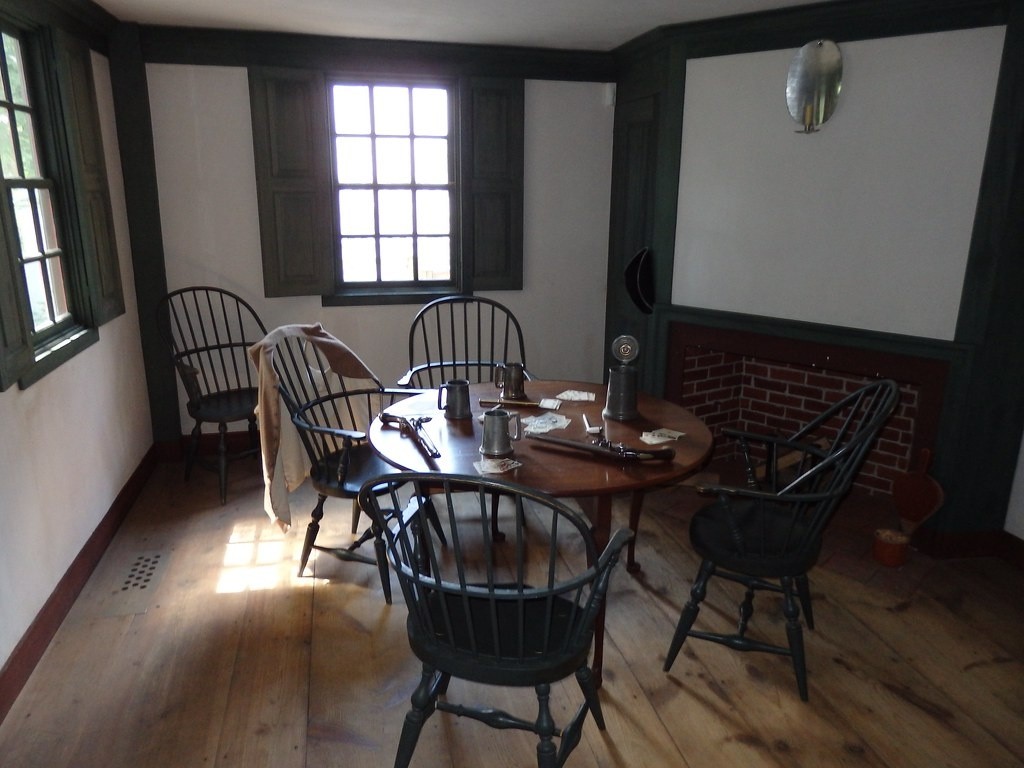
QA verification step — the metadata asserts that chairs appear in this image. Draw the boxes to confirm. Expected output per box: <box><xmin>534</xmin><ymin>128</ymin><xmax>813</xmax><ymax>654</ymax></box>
<box><xmin>155</xmin><ymin>287</ymin><xmax>268</xmax><ymax>505</ymax></box>
<box><xmin>397</xmin><ymin>296</ymin><xmax>542</xmax><ymax>527</ymax></box>
<box><xmin>661</xmin><ymin>380</ymin><xmax>902</xmax><ymax>703</ymax></box>
<box><xmin>356</xmin><ymin>473</ymin><xmax>636</xmax><ymax>768</ymax></box>
<box><xmin>248</xmin><ymin>322</ymin><xmax>448</xmax><ymax>606</ymax></box>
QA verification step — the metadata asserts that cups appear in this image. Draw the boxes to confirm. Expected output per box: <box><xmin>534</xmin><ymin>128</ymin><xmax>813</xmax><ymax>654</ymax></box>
<box><xmin>479</xmin><ymin>411</ymin><xmax>520</xmax><ymax>456</ymax></box>
<box><xmin>601</xmin><ymin>335</ymin><xmax>641</xmax><ymax>422</ymax></box>
<box><xmin>438</xmin><ymin>379</ymin><xmax>473</xmax><ymax>420</ymax></box>
<box><xmin>495</xmin><ymin>362</ymin><xmax>528</xmax><ymax>400</ymax></box>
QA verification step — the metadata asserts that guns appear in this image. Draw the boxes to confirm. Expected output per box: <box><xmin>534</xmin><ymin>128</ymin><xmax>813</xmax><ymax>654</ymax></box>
<box><xmin>526</xmin><ymin>431</ymin><xmax>676</xmax><ymax>465</ymax></box>
<box><xmin>377</xmin><ymin>412</ymin><xmax>440</xmax><ymax>473</ymax></box>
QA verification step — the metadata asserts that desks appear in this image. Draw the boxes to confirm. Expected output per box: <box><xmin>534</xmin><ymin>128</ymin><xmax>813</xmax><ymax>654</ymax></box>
<box><xmin>367</xmin><ymin>380</ymin><xmax>712</xmax><ymax>691</ymax></box>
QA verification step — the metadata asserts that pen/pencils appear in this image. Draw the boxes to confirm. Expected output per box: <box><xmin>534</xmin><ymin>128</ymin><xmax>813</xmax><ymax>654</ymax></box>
<box><xmin>478</xmin><ymin>399</ymin><xmax>539</xmax><ymax>408</ymax></box>
